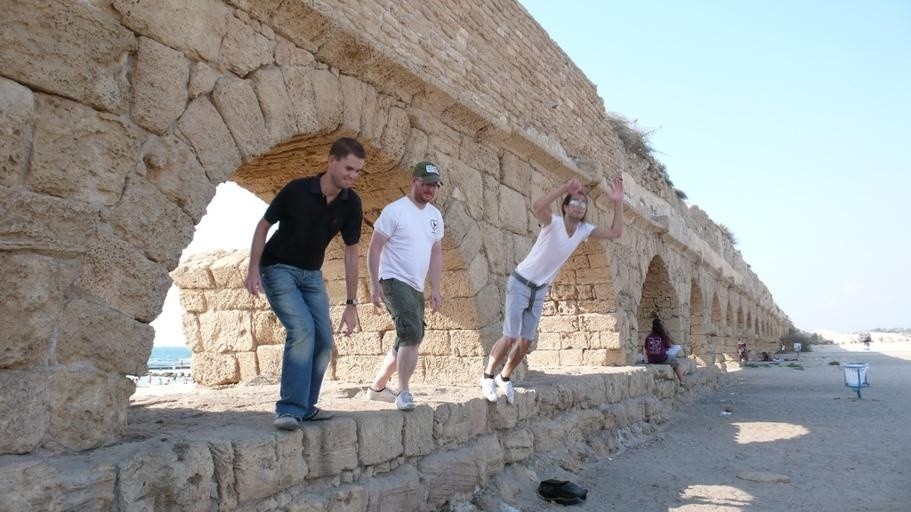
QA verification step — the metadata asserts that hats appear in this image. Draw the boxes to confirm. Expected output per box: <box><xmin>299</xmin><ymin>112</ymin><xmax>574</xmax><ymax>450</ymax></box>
<box><xmin>413</xmin><ymin>161</ymin><xmax>440</xmax><ymax>184</ymax></box>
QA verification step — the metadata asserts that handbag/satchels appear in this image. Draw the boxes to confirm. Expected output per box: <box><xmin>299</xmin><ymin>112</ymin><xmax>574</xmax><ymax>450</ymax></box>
<box><xmin>539</xmin><ymin>479</ymin><xmax>587</xmax><ymax>505</ymax></box>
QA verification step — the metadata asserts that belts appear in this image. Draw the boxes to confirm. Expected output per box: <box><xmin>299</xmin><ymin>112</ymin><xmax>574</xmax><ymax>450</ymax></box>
<box><xmin>512</xmin><ymin>270</ymin><xmax>547</xmax><ymax>311</ymax></box>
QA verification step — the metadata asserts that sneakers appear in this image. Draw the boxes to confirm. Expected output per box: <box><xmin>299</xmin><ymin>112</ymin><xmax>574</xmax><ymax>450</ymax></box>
<box><xmin>367</xmin><ymin>387</ymin><xmax>398</xmax><ymax>402</ymax></box>
<box><xmin>274</xmin><ymin>414</ymin><xmax>298</xmax><ymax>430</ymax></box>
<box><xmin>303</xmin><ymin>408</ymin><xmax>334</xmax><ymax>419</ymax></box>
<box><xmin>479</xmin><ymin>374</ymin><xmax>497</xmax><ymax>402</ymax></box>
<box><xmin>396</xmin><ymin>392</ymin><xmax>416</xmax><ymax>410</ymax></box>
<box><xmin>495</xmin><ymin>374</ymin><xmax>514</xmax><ymax>405</ymax></box>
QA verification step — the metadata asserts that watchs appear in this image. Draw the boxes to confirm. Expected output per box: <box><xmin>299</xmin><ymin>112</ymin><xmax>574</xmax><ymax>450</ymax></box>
<box><xmin>346</xmin><ymin>296</ymin><xmax>360</xmax><ymax>306</ymax></box>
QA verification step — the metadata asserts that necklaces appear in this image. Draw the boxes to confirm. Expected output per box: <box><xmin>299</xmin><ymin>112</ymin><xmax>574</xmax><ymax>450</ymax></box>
<box><xmin>565</xmin><ymin>216</ymin><xmax>579</xmax><ymax>237</ymax></box>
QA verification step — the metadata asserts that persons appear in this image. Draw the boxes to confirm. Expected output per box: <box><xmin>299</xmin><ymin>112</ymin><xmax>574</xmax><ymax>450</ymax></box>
<box><xmin>368</xmin><ymin>159</ymin><xmax>448</xmax><ymax>411</ymax></box>
<box><xmin>474</xmin><ymin>176</ymin><xmax>625</xmax><ymax>404</ymax></box>
<box><xmin>738</xmin><ymin>343</ymin><xmax>747</xmax><ymax>366</ymax></box>
<box><xmin>761</xmin><ymin>351</ymin><xmax>800</xmax><ymax>362</ymax></box>
<box><xmin>643</xmin><ymin>318</ymin><xmax>690</xmax><ymax>391</ymax></box>
<box><xmin>243</xmin><ymin>136</ymin><xmax>368</xmax><ymax>432</ymax></box>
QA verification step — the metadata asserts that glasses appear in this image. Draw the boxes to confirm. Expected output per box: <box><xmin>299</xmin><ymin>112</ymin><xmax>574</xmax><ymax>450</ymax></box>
<box><xmin>570</xmin><ymin>199</ymin><xmax>586</xmax><ymax>208</ymax></box>
